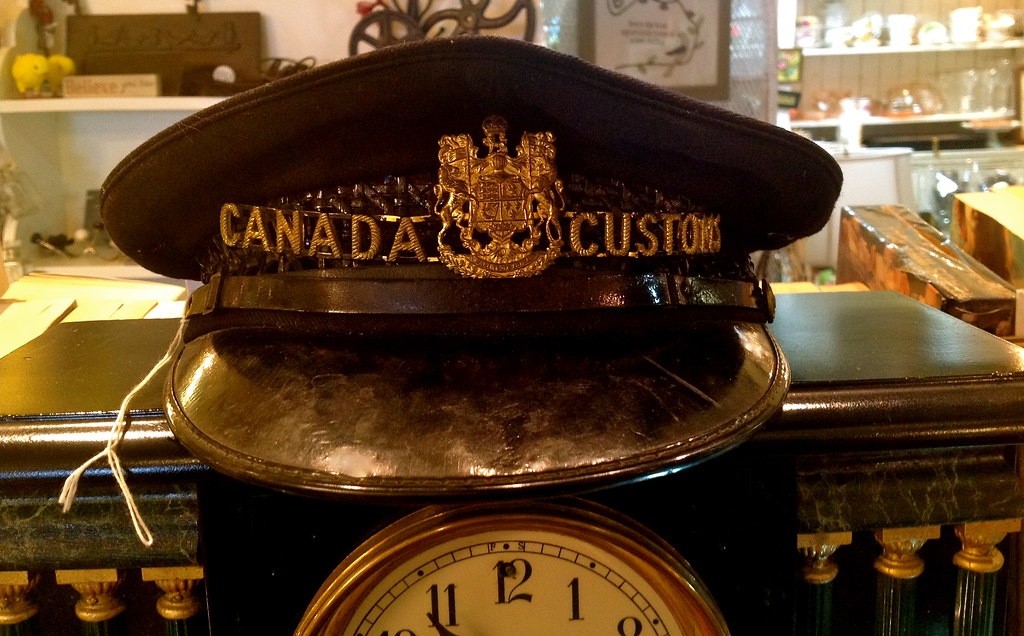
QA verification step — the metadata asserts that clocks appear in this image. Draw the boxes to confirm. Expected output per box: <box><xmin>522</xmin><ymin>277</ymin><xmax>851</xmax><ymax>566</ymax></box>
<box><xmin>294</xmin><ymin>494</ymin><xmax>732</xmax><ymax>636</ymax></box>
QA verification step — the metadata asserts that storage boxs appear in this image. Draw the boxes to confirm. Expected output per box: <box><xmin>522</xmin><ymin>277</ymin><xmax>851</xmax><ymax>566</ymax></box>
<box><xmin>837</xmin><ymin>204</ymin><xmax>1017</xmax><ymax>337</ymax></box>
<box><xmin>62</xmin><ymin>73</ymin><xmax>162</xmax><ymax>98</ymax></box>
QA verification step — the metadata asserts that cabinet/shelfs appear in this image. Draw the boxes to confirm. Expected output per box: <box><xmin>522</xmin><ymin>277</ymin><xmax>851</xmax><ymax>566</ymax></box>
<box><xmin>774</xmin><ymin>0</ymin><xmax>1024</xmax><ymax>128</ymax></box>
<box><xmin>0</xmin><ymin>6</ymin><xmax>233</xmax><ymax>280</ymax></box>
<box><xmin>869</xmin><ymin>134</ymin><xmax>1024</xmax><ymax>236</ymax></box>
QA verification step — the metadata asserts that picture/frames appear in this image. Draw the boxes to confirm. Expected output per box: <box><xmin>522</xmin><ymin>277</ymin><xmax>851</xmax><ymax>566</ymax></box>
<box><xmin>579</xmin><ymin>0</ymin><xmax>731</xmax><ymax>100</ymax></box>
